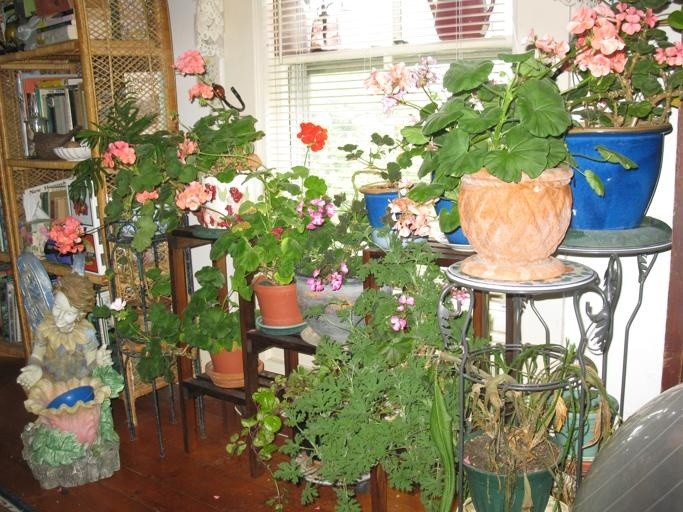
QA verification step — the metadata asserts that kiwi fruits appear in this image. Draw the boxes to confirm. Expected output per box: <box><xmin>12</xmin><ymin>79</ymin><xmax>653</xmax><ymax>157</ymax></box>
<box><xmin>63</xmin><ymin>142</ymin><xmax>80</xmax><ymax>148</ymax></box>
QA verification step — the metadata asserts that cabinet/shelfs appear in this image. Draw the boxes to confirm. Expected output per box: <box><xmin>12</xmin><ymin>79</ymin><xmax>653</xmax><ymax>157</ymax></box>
<box><xmin>0</xmin><ymin>0</ymin><xmax>191</xmax><ymax>428</ymax></box>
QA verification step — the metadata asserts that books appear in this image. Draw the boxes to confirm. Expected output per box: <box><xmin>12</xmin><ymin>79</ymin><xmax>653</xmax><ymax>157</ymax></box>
<box><xmin>22</xmin><ymin>177</ymin><xmax>73</xmax><ymax>231</ymax></box>
<box><xmin>6</xmin><ymin>283</ymin><xmax>22</xmax><ymax>344</ymax></box>
<box><xmin>95</xmin><ymin>288</ymin><xmax>113</xmax><ymax>348</ymax></box>
<box><xmin>24</xmin><ymin>9</ymin><xmax>111</xmax><ymax>49</ymax></box>
<box><xmin>16</xmin><ymin>71</ymin><xmax>166</xmax><ymax>158</ymax></box>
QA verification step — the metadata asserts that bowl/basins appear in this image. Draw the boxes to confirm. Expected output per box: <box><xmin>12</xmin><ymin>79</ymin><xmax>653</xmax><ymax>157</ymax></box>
<box><xmin>49</xmin><ymin>385</ymin><xmax>94</xmax><ymax>409</ymax></box>
<box><xmin>52</xmin><ymin>148</ymin><xmax>91</xmax><ymax>162</ymax></box>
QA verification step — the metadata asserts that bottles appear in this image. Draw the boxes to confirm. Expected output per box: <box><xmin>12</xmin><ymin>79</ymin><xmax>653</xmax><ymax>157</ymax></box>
<box><xmin>25</xmin><ymin>112</ymin><xmax>48</xmax><ymax>159</ymax></box>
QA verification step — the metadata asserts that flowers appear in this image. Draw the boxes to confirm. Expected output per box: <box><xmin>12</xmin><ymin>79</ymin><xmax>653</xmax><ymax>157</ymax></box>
<box><xmin>366</xmin><ymin>270</ymin><xmax>481</xmax><ymax>347</ymax></box>
<box><xmin>361</xmin><ymin>51</ymin><xmax>456</xmax><ymax>130</ymax></box>
<box><xmin>49</xmin><ymin>48</ymin><xmax>258</xmax><ymax>266</ymax></box>
<box><xmin>529</xmin><ymin>0</ymin><xmax>683</xmax><ymax>127</ymax></box>
<box><xmin>222</xmin><ymin>118</ymin><xmax>353</xmax><ymax>294</ymax></box>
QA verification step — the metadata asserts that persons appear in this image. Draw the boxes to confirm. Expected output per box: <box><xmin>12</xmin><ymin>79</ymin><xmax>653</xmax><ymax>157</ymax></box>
<box><xmin>17</xmin><ymin>274</ymin><xmax>115</xmax><ymax>391</ymax></box>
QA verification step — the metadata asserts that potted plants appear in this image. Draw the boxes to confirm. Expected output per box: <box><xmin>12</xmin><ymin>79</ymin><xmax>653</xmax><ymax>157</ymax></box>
<box><xmin>454</xmin><ymin>355</ymin><xmax>568</xmax><ymax>511</ymax></box>
<box><xmin>421</xmin><ymin>48</ymin><xmax>577</xmax><ymax>282</ymax></box>
<box><xmin>150</xmin><ymin>267</ymin><xmax>260</xmax><ymax>388</ymax></box>
<box><xmin>345</xmin><ymin>131</ymin><xmax>418</xmax><ymax>229</ymax></box>
